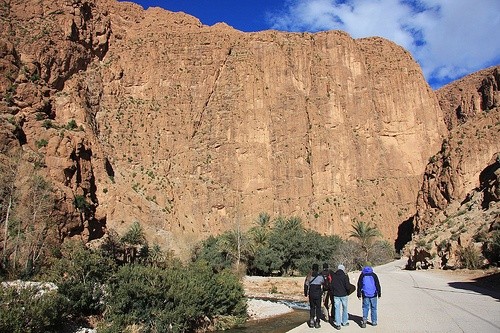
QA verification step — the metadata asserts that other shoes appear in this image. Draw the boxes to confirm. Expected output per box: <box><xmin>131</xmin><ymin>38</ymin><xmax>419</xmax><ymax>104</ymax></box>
<box><xmin>372</xmin><ymin>323</ymin><xmax>377</xmax><ymax>326</ymax></box>
<box><xmin>342</xmin><ymin>322</ymin><xmax>349</xmax><ymax>326</ymax></box>
<box><xmin>336</xmin><ymin>325</ymin><xmax>341</xmax><ymax>330</ymax></box>
<box><xmin>307</xmin><ymin>320</ymin><xmax>321</xmax><ymax>328</ymax></box>
<box><xmin>361</xmin><ymin>319</ymin><xmax>368</xmax><ymax>325</ymax></box>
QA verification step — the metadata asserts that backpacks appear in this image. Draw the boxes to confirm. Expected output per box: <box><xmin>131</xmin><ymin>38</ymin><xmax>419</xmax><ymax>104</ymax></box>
<box><xmin>322</xmin><ymin>274</ymin><xmax>330</xmax><ymax>291</ymax></box>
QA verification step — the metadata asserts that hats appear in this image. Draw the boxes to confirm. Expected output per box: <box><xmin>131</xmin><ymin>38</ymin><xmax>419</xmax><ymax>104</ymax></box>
<box><xmin>363</xmin><ymin>261</ymin><xmax>373</xmax><ymax>267</ymax></box>
<box><xmin>338</xmin><ymin>264</ymin><xmax>346</xmax><ymax>272</ymax></box>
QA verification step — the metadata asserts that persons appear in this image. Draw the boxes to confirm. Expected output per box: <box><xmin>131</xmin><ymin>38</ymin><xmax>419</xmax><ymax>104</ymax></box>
<box><xmin>305</xmin><ymin>263</ymin><xmax>323</xmax><ymax>327</ymax></box>
<box><xmin>330</xmin><ymin>264</ymin><xmax>356</xmax><ymax>330</ymax></box>
<box><xmin>357</xmin><ymin>261</ymin><xmax>382</xmax><ymax>328</ymax></box>
<box><xmin>320</xmin><ymin>263</ymin><xmax>335</xmax><ymax>323</ymax></box>
